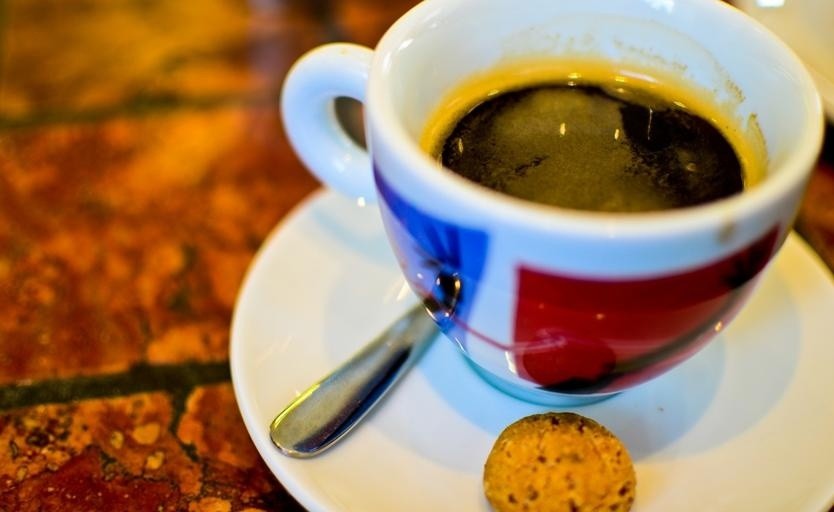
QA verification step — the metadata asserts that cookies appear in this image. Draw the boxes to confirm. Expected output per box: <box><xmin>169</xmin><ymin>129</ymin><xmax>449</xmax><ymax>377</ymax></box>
<box><xmin>483</xmin><ymin>412</ymin><xmax>636</xmax><ymax>512</ymax></box>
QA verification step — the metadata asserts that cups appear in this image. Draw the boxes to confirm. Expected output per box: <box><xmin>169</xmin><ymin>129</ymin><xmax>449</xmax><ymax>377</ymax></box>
<box><xmin>276</xmin><ymin>0</ymin><xmax>828</xmax><ymax>411</ymax></box>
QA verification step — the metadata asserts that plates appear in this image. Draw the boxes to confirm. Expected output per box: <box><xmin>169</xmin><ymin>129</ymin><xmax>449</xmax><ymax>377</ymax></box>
<box><xmin>225</xmin><ymin>182</ymin><xmax>834</xmax><ymax>512</ymax></box>
